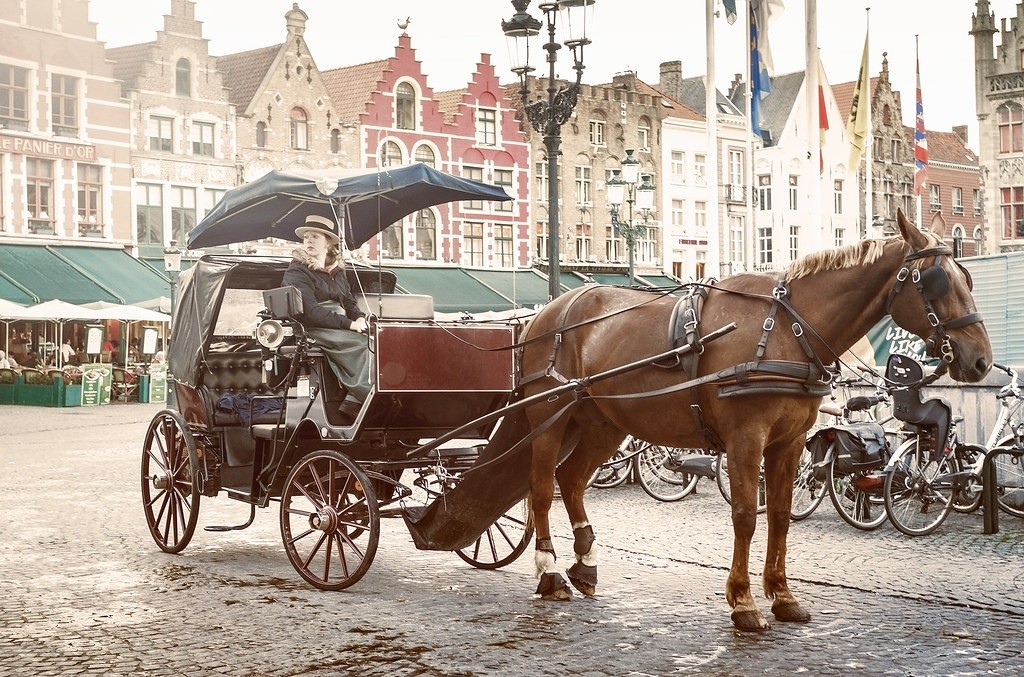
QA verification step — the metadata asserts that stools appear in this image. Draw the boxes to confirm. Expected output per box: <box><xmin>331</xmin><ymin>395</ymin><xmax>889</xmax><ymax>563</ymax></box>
<box><xmin>252</xmin><ymin>424</ymin><xmax>284</xmax><ymax>440</ymax></box>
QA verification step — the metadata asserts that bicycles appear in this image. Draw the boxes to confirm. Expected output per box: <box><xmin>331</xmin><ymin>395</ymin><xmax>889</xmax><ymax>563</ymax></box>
<box><xmin>548</xmin><ymin>361</ymin><xmax>985</xmax><ymax>537</ymax></box>
<box><xmin>881</xmin><ymin>363</ymin><xmax>1024</xmax><ymax>536</ymax></box>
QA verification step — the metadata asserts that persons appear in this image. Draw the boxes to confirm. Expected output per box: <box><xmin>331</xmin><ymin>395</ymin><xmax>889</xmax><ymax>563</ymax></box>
<box><xmin>48</xmin><ymin>337</ymin><xmax>89</xmax><ymax>367</ymax></box>
<box><xmin>102</xmin><ymin>337</ymin><xmax>139</xmax><ymax>352</ymax></box>
<box><xmin>281</xmin><ymin>215</ymin><xmax>375</xmax><ymax>428</ymax></box>
<box><xmin>0</xmin><ymin>349</ymin><xmax>19</xmax><ymax>368</ymax></box>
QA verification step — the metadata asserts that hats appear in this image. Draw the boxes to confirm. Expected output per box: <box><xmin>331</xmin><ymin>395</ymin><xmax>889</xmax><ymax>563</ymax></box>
<box><xmin>294</xmin><ymin>215</ymin><xmax>339</xmax><ymax>245</ymax></box>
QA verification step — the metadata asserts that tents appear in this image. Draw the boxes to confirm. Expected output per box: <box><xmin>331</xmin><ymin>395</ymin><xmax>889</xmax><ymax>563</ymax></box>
<box><xmin>0</xmin><ymin>295</ymin><xmax>173</xmax><ymax>403</ymax></box>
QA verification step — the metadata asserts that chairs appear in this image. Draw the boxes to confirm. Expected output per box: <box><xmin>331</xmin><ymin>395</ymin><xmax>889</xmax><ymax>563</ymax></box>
<box><xmin>0</xmin><ymin>351</ymin><xmax>138</xmax><ymax>404</ymax></box>
<box><xmin>884</xmin><ymin>353</ymin><xmax>952</xmax><ymax>462</ymax></box>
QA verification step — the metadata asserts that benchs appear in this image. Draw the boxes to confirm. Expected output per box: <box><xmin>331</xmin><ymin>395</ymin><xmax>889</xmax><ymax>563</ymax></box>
<box><xmin>200</xmin><ymin>353</ymin><xmax>316</xmax><ymax>425</ymax></box>
<box><xmin>261</xmin><ymin>287</ymin><xmax>434</xmax><ymax>347</ymax></box>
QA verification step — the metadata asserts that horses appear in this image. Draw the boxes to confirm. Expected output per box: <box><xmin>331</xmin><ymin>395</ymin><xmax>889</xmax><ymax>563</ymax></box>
<box><xmin>516</xmin><ymin>207</ymin><xmax>993</xmax><ymax>633</ymax></box>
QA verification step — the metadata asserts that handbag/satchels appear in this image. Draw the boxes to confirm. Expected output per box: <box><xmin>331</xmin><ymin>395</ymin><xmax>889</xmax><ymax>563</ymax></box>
<box><xmin>806</xmin><ymin>421</ymin><xmax>889</xmax><ymax>480</ymax></box>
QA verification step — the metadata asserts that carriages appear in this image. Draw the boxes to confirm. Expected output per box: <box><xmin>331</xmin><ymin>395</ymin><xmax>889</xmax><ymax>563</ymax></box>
<box><xmin>139</xmin><ymin>162</ymin><xmax>994</xmax><ymax>634</ymax></box>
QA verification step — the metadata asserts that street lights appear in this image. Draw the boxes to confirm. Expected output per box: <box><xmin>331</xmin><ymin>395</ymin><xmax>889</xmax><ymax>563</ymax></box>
<box><xmin>604</xmin><ymin>148</ymin><xmax>657</xmax><ymax>287</ymax></box>
<box><xmin>501</xmin><ymin>0</ymin><xmax>595</xmax><ymax>302</ymax></box>
<box><xmin>163</xmin><ymin>238</ymin><xmax>182</xmax><ymax>333</ymax></box>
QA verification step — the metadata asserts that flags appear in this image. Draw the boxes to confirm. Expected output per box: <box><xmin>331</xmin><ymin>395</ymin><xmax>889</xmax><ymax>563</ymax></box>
<box><xmin>723</xmin><ymin>0</ymin><xmax>738</xmax><ymax>25</ymax></box>
<box><xmin>914</xmin><ymin>54</ymin><xmax>928</xmax><ymax>197</ymax></box>
<box><xmin>846</xmin><ymin>30</ymin><xmax>868</xmax><ymax>174</ymax></box>
<box><xmin>817</xmin><ymin>59</ymin><xmax>829</xmax><ymax>176</ymax></box>
<box><xmin>750</xmin><ymin>0</ymin><xmax>785</xmax><ymax>141</ymax></box>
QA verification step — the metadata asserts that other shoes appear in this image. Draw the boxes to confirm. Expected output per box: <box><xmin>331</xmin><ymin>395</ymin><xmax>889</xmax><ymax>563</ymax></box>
<box><xmin>338</xmin><ymin>401</ymin><xmax>362</xmax><ymax>419</ymax></box>
